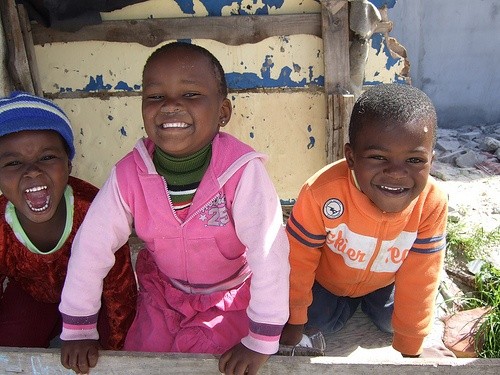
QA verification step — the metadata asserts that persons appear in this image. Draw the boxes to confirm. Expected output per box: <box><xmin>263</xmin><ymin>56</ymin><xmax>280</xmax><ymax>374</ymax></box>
<box><xmin>278</xmin><ymin>83</ymin><xmax>448</xmax><ymax>358</ymax></box>
<box><xmin>0</xmin><ymin>90</ymin><xmax>138</xmax><ymax>350</ymax></box>
<box><xmin>59</xmin><ymin>42</ymin><xmax>291</xmax><ymax>375</ymax></box>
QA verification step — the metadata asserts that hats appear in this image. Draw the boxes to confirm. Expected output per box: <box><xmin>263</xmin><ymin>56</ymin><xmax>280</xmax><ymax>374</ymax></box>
<box><xmin>0</xmin><ymin>90</ymin><xmax>76</xmax><ymax>161</ymax></box>
